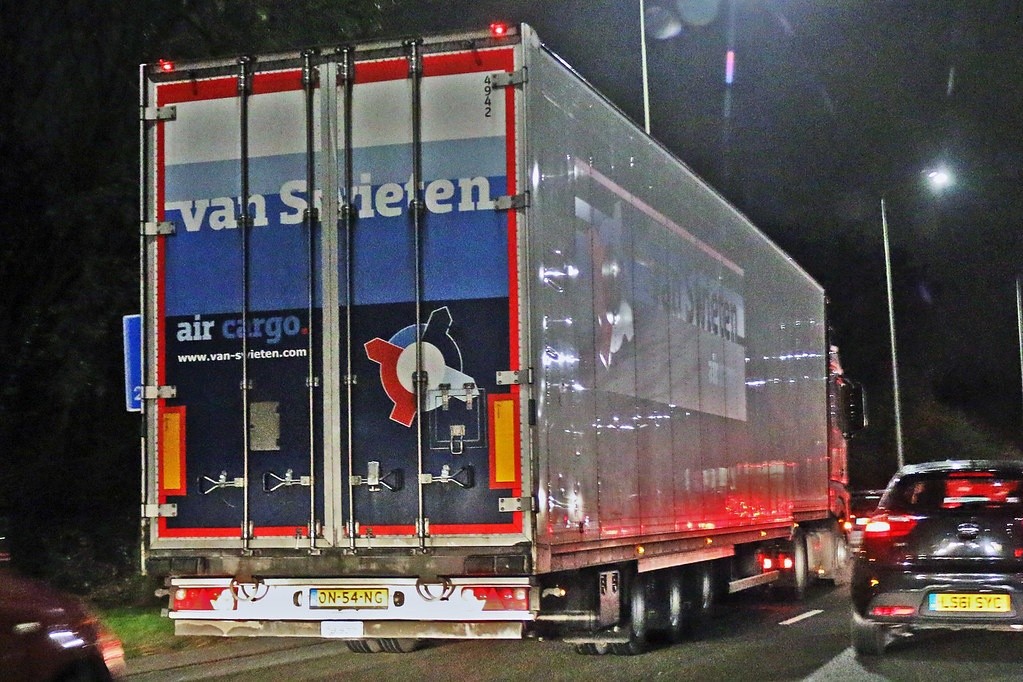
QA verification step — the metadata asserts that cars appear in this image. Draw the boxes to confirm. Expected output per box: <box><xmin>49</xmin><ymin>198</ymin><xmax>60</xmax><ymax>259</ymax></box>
<box><xmin>839</xmin><ymin>488</ymin><xmax>889</xmax><ymax>547</ymax></box>
<box><xmin>0</xmin><ymin>550</ymin><xmax>127</xmax><ymax>682</ymax></box>
<box><xmin>849</xmin><ymin>458</ymin><xmax>1023</xmax><ymax>660</ymax></box>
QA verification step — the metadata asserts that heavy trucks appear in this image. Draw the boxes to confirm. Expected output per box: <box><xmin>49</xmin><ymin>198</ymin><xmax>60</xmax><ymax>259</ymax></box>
<box><xmin>137</xmin><ymin>22</ymin><xmax>870</xmax><ymax>658</ymax></box>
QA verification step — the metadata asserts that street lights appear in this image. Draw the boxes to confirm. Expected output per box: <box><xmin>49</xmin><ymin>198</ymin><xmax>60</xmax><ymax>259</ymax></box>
<box><xmin>879</xmin><ymin>160</ymin><xmax>957</xmax><ymax>471</ymax></box>
